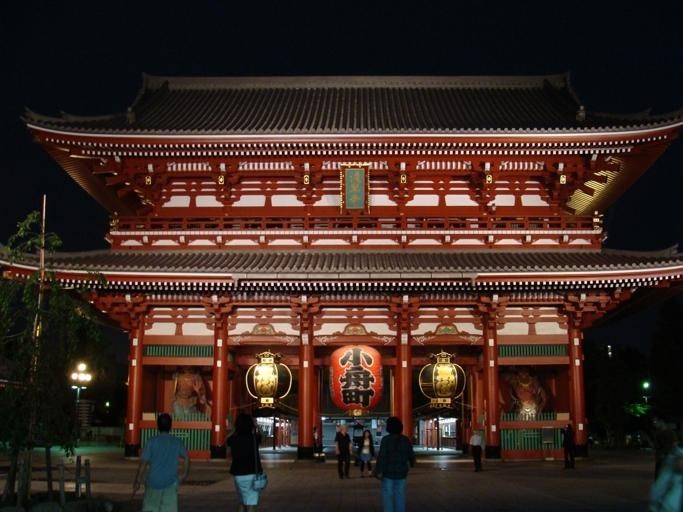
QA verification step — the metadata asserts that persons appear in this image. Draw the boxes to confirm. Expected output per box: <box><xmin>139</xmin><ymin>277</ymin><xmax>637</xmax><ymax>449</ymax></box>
<box><xmin>509</xmin><ymin>365</ymin><xmax>548</xmax><ymax>419</ymax></box>
<box><xmin>648</xmin><ymin>421</ymin><xmax>683</xmax><ymax>512</ymax></box>
<box><xmin>334</xmin><ymin>425</ymin><xmax>353</xmax><ymax>480</ymax></box>
<box><xmin>563</xmin><ymin>424</ymin><xmax>575</xmax><ymax>470</ymax></box>
<box><xmin>651</xmin><ymin>414</ymin><xmax>675</xmax><ymax>481</ymax></box>
<box><xmin>370</xmin><ymin>417</ymin><xmax>417</xmax><ymax>512</ymax></box>
<box><xmin>470</xmin><ymin>430</ymin><xmax>482</xmax><ymax>472</ymax></box>
<box><xmin>357</xmin><ymin>430</ymin><xmax>375</xmax><ymax>477</ymax></box>
<box><xmin>134</xmin><ymin>413</ymin><xmax>190</xmax><ymax>512</ymax></box>
<box><xmin>171</xmin><ymin>366</ymin><xmax>206</xmax><ymax>416</ymax></box>
<box><xmin>227</xmin><ymin>413</ymin><xmax>265</xmax><ymax>512</ymax></box>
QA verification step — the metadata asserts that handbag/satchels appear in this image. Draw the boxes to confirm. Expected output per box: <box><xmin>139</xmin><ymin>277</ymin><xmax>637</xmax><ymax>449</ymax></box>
<box><xmin>252</xmin><ymin>474</ymin><xmax>267</xmax><ymax>490</ymax></box>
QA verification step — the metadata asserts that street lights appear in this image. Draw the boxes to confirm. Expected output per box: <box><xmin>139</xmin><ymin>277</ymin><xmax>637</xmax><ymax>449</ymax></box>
<box><xmin>71</xmin><ymin>363</ymin><xmax>92</xmax><ymax>448</ymax></box>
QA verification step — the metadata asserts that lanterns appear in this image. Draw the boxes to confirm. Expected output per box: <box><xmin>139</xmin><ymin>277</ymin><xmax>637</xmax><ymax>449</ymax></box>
<box><xmin>418</xmin><ymin>352</ymin><xmax>466</xmax><ymax>410</ymax></box>
<box><xmin>329</xmin><ymin>344</ymin><xmax>384</xmax><ymax>417</ymax></box>
<box><xmin>245</xmin><ymin>352</ymin><xmax>292</xmax><ymax>409</ymax></box>
